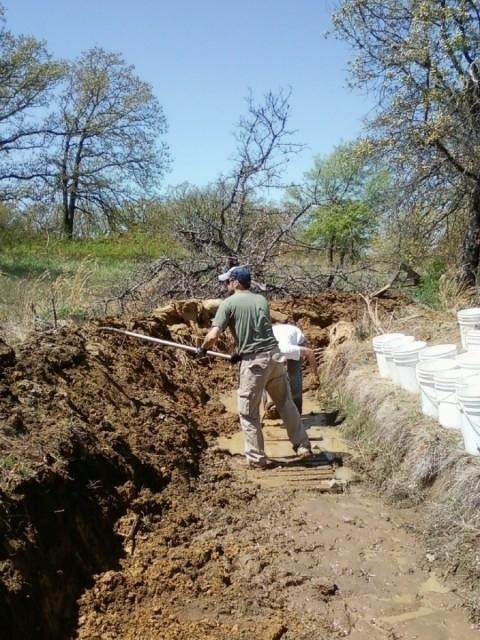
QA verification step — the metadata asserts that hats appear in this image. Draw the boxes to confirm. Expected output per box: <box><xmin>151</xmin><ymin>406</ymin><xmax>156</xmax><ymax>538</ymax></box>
<box><xmin>218</xmin><ymin>267</ymin><xmax>250</xmax><ymax>284</ymax></box>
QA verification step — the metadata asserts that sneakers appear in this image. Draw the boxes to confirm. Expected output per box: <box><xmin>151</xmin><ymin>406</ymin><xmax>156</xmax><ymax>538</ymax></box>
<box><xmin>238</xmin><ymin>460</ymin><xmax>265</xmax><ymax>470</ymax></box>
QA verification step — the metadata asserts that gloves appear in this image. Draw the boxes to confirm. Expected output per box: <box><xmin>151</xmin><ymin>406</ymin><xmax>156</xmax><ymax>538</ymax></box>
<box><xmin>230</xmin><ymin>354</ymin><xmax>241</xmax><ymax>364</ymax></box>
<box><xmin>197</xmin><ymin>346</ymin><xmax>207</xmax><ymax>358</ymax></box>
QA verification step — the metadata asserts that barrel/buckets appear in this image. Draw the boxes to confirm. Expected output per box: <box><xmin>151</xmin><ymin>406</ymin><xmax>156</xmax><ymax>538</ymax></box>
<box><xmin>369</xmin><ymin>308</ymin><xmax>480</xmax><ymax>456</ymax></box>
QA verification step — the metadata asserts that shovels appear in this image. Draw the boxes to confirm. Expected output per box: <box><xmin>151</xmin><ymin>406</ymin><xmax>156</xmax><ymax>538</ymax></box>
<box><xmin>69</xmin><ymin>314</ymin><xmax>232</xmax><ymax>361</ymax></box>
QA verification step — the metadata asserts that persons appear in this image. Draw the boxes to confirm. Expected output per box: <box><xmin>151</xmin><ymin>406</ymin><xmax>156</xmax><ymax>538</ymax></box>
<box><xmin>195</xmin><ymin>266</ymin><xmax>313</xmax><ymax>470</ymax></box>
<box><xmin>271</xmin><ymin>323</ymin><xmax>320</xmax><ymax>415</ymax></box>
